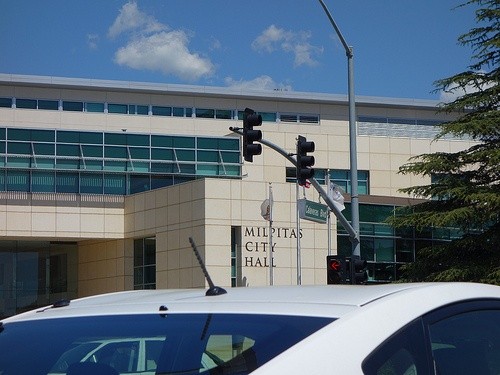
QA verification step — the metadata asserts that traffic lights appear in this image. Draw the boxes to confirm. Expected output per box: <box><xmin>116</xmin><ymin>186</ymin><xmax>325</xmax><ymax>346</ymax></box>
<box><xmin>349</xmin><ymin>255</ymin><xmax>368</xmax><ymax>285</ymax></box>
<box><xmin>327</xmin><ymin>254</ymin><xmax>347</xmax><ymax>284</ymax></box>
<box><xmin>241</xmin><ymin>107</ymin><xmax>263</xmax><ymax>162</ymax></box>
<box><xmin>295</xmin><ymin>135</ymin><xmax>315</xmax><ymax>186</ymax></box>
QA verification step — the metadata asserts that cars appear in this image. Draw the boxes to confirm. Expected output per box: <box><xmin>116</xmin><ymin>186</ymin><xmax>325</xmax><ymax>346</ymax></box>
<box><xmin>0</xmin><ymin>285</ymin><xmax>500</xmax><ymax>375</ymax></box>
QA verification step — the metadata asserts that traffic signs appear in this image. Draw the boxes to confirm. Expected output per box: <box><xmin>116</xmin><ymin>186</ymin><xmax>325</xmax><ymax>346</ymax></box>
<box><xmin>298</xmin><ymin>198</ymin><xmax>328</xmax><ymax>224</ymax></box>
